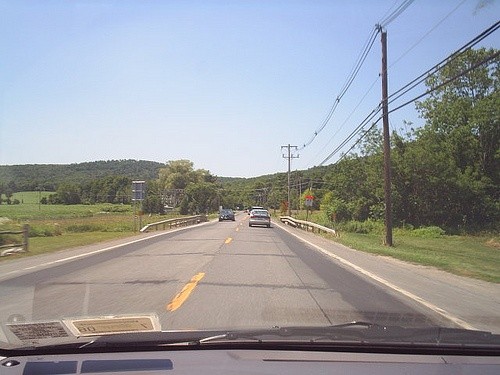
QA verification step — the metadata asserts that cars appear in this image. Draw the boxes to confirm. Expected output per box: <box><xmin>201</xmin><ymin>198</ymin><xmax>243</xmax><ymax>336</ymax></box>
<box><xmin>248</xmin><ymin>208</ymin><xmax>271</xmax><ymax>227</ymax></box>
<box><xmin>218</xmin><ymin>208</ymin><xmax>234</xmax><ymax>221</ymax></box>
